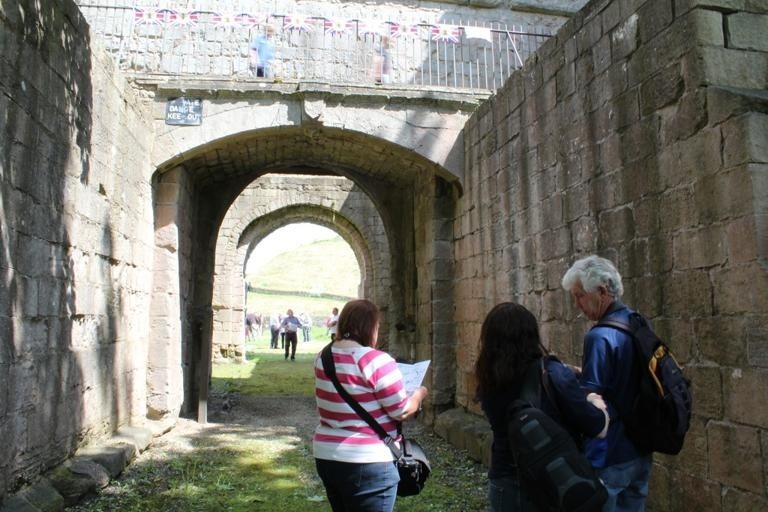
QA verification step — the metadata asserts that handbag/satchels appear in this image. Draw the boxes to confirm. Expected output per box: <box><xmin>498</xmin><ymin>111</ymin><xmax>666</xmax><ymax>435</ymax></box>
<box><xmin>389</xmin><ymin>434</ymin><xmax>432</xmax><ymax>498</ymax></box>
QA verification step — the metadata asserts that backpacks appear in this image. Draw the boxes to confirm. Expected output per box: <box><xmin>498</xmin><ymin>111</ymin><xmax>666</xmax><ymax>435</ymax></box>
<box><xmin>589</xmin><ymin>311</ymin><xmax>695</xmax><ymax>458</ymax></box>
<box><xmin>495</xmin><ymin>354</ymin><xmax>611</xmax><ymax>511</ymax></box>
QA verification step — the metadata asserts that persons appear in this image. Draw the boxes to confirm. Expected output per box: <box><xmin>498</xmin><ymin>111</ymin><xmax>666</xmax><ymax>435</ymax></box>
<box><xmin>472</xmin><ymin>302</ymin><xmax>609</xmax><ymax>510</ymax></box>
<box><xmin>559</xmin><ymin>253</ymin><xmax>692</xmax><ymax>512</ymax></box>
<box><xmin>244</xmin><ymin>312</ymin><xmax>312</xmax><ymax>350</ymax></box>
<box><xmin>275</xmin><ymin>309</ymin><xmax>302</xmax><ymax>361</ymax></box>
<box><xmin>312</xmin><ymin>297</ymin><xmax>430</xmax><ymax>512</ymax></box>
<box><xmin>327</xmin><ymin>307</ymin><xmax>339</xmax><ymax>341</ymax></box>
